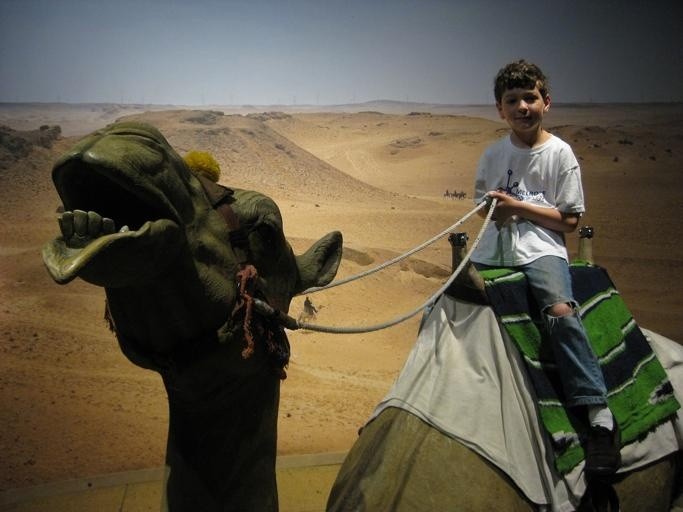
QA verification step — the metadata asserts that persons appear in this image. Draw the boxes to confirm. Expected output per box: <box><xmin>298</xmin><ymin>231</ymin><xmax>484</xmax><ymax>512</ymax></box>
<box><xmin>467</xmin><ymin>59</ymin><xmax>625</xmax><ymax>479</ymax></box>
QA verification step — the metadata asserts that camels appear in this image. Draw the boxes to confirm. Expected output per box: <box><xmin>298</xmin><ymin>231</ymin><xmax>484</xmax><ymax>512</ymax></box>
<box><xmin>40</xmin><ymin>119</ymin><xmax>682</xmax><ymax>511</ymax></box>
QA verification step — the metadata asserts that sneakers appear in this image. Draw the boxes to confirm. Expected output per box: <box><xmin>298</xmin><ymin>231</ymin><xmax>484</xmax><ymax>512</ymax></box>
<box><xmin>585</xmin><ymin>413</ymin><xmax>622</xmax><ymax>476</ymax></box>
